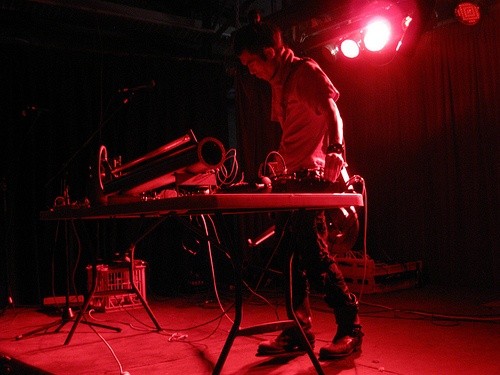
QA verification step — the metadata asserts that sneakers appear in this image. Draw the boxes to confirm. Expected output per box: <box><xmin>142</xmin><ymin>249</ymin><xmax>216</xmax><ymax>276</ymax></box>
<box><xmin>319</xmin><ymin>334</ymin><xmax>363</xmax><ymax>357</ymax></box>
<box><xmin>256</xmin><ymin>333</ymin><xmax>315</xmax><ymax>356</ymax></box>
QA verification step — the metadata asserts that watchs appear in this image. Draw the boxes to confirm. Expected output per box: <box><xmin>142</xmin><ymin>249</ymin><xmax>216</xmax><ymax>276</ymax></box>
<box><xmin>327</xmin><ymin>143</ymin><xmax>345</xmax><ymax>155</ymax></box>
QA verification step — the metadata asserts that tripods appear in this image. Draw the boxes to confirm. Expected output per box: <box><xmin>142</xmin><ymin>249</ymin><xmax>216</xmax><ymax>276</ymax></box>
<box><xmin>15</xmin><ymin>220</ymin><xmax>121</xmax><ymax>340</ymax></box>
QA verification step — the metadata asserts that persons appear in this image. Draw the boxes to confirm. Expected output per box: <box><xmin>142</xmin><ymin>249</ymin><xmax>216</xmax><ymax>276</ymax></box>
<box><xmin>230</xmin><ymin>21</ymin><xmax>365</xmax><ymax>359</ymax></box>
